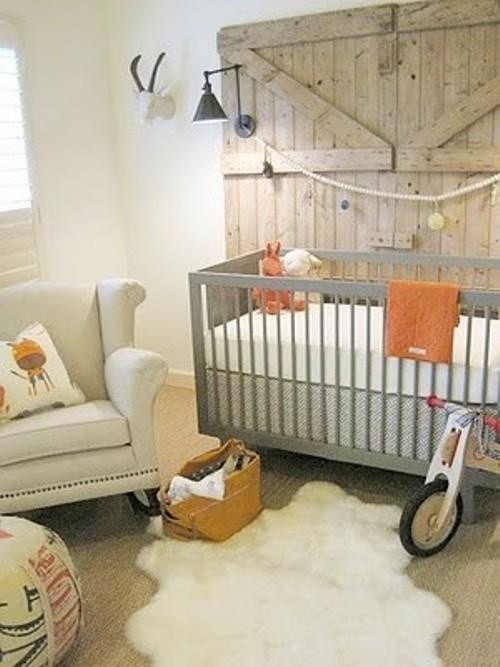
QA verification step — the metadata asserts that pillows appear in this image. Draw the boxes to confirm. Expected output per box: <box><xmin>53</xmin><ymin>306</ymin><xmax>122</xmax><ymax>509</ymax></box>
<box><xmin>1</xmin><ymin>321</ymin><xmax>85</xmax><ymax>424</ymax></box>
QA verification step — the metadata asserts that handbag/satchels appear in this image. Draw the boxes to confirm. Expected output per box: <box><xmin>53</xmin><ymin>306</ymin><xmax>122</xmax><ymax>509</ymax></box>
<box><xmin>157</xmin><ymin>438</ymin><xmax>264</xmax><ymax>543</ymax></box>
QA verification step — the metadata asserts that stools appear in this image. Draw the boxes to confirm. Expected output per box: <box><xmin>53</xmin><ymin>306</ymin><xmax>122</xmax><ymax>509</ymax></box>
<box><xmin>0</xmin><ymin>515</ymin><xmax>83</xmax><ymax>665</ymax></box>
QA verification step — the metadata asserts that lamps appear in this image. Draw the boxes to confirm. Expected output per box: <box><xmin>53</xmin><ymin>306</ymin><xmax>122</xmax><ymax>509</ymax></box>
<box><xmin>192</xmin><ymin>64</ymin><xmax>256</xmax><ymax>137</ymax></box>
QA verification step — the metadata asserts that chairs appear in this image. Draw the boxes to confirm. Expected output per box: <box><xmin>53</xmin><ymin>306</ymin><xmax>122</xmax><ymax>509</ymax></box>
<box><xmin>1</xmin><ymin>273</ymin><xmax>167</xmax><ymax>516</ymax></box>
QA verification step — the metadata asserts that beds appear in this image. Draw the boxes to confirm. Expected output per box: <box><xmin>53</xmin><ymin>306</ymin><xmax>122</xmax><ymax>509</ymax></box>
<box><xmin>188</xmin><ymin>243</ymin><xmax>500</xmax><ymax>524</ymax></box>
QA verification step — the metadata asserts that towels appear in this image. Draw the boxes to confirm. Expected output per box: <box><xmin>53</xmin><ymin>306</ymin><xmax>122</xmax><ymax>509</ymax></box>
<box><xmin>382</xmin><ymin>279</ymin><xmax>460</xmax><ymax>364</ymax></box>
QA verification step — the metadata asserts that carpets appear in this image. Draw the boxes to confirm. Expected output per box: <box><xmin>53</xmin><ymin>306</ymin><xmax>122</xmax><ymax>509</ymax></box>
<box><xmin>125</xmin><ymin>481</ymin><xmax>453</xmax><ymax>664</ymax></box>
